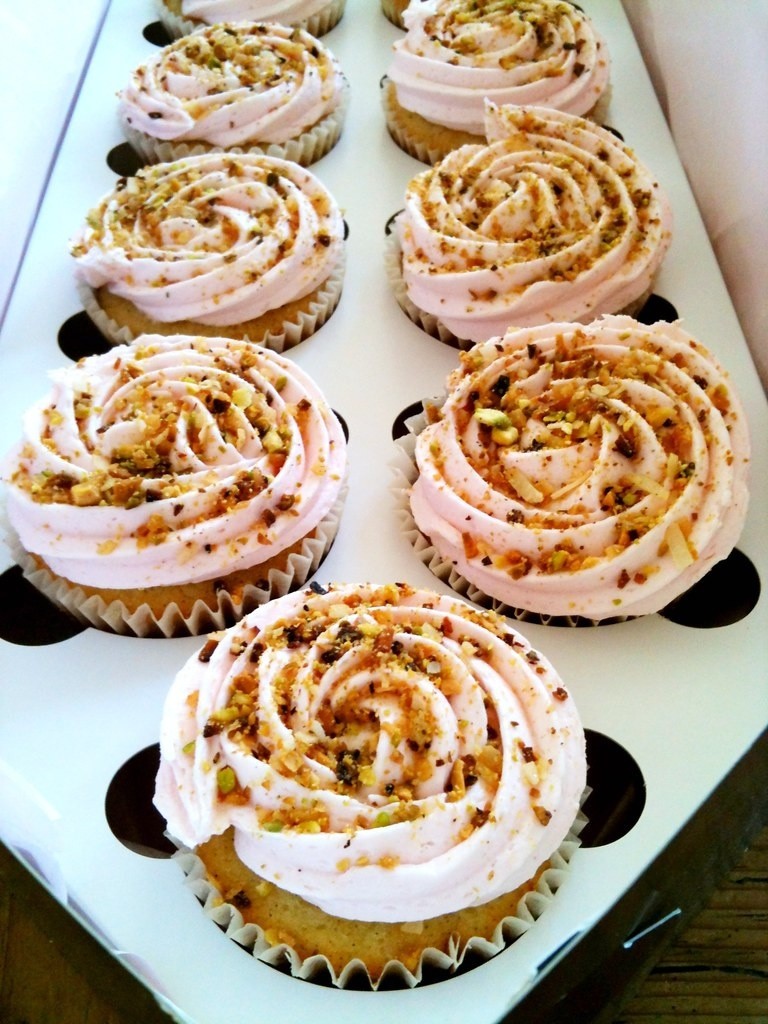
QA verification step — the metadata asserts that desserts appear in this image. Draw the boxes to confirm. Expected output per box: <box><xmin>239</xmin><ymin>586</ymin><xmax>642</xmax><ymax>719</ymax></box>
<box><xmin>0</xmin><ymin>0</ymin><xmax>752</xmax><ymax>988</ymax></box>
